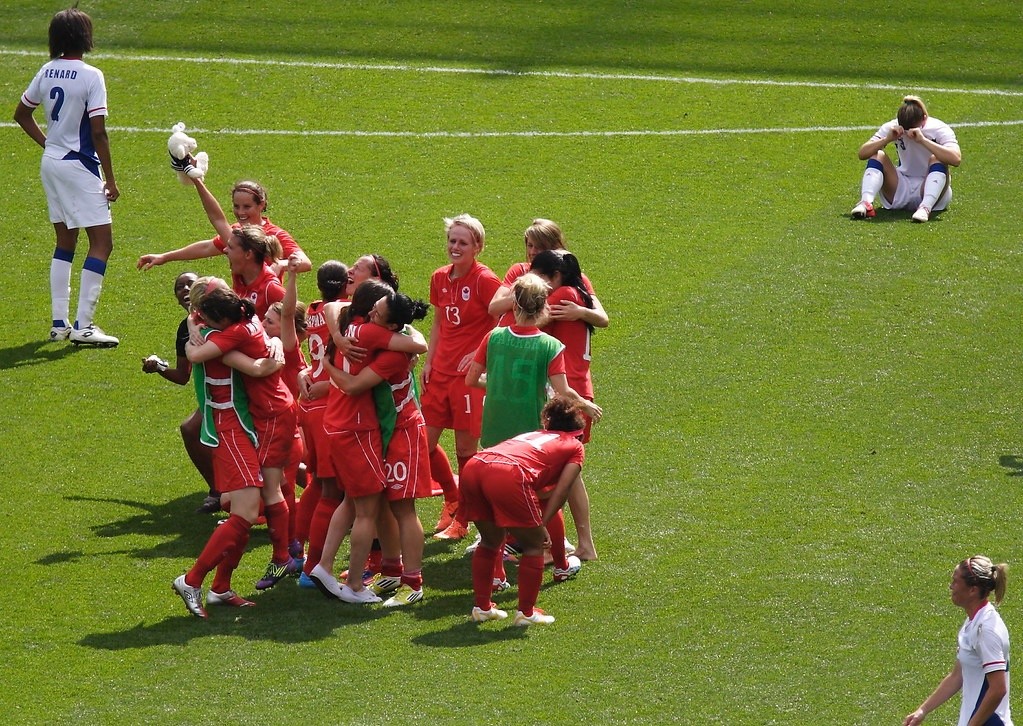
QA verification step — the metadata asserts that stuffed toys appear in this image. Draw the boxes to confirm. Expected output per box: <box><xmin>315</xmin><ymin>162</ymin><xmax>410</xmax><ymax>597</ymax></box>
<box><xmin>168</xmin><ymin>122</ymin><xmax>209</xmax><ymax>184</ymax></box>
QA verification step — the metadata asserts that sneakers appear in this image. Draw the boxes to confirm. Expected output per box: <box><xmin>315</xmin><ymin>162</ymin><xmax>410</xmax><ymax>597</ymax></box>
<box><xmin>912</xmin><ymin>208</ymin><xmax>929</xmax><ymax>222</ymax></box>
<box><xmin>67</xmin><ymin>327</ymin><xmax>120</xmax><ymax>349</ymax></box>
<box><xmin>513</xmin><ymin>609</ymin><xmax>555</xmax><ymax>627</ymax></box>
<box><xmin>171</xmin><ymin>573</ymin><xmax>208</xmax><ymax>619</ymax></box>
<box><xmin>367</xmin><ymin>573</ymin><xmax>403</xmax><ymax>595</ymax></box>
<box><xmin>466</xmin><ymin>534</ymin><xmax>483</xmax><ymax>553</ymax></box>
<box><xmin>553</xmin><ymin>555</ymin><xmax>581</xmax><ymax>581</ymax></box>
<box><xmin>472</xmin><ymin>607</ymin><xmax>508</xmax><ymax>622</ymax></box>
<box><xmin>435</xmin><ymin>501</ymin><xmax>459</xmax><ymax>531</ymax></box>
<box><xmin>851</xmin><ymin>201</ymin><xmax>874</xmax><ymax>219</ymax></box>
<box><xmin>255</xmin><ymin>555</ymin><xmax>297</xmax><ymax>589</ymax></box>
<box><xmin>206</xmin><ymin>589</ymin><xmax>256</xmax><ymax>607</ymax></box>
<box><xmin>382</xmin><ymin>583</ymin><xmax>423</xmax><ymax>609</ymax></box>
<box><xmin>195</xmin><ymin>495</ymin><xmax>223</xmax><ymax>512</ymax></box>
<box><xmin>337</xmin><ymin>583</ymin><xmax>384</xmax><ymax>604</ymax></box>
<box><xmin>310</xmin><ymin>564</ymin><xmax>340</xmax><ymax>597</ymax></box>
<box><xmin>491</xmin><ymin>578</ymin><xmax>510</xmax><ymax>591</ymax></box>
<box><xmin>52</xmin><ymin>325</ymin><xmax>71</xmax><ymax>339</ymax></box>
<box><xmin>433</xmin><ymin>519</ymin><xmax>471</xmax><ymax>540</ymax></box>
<box><xmin>288</xmin><ymin>540</ymin><xmax>303</xmax><ymax>558</ymax></box>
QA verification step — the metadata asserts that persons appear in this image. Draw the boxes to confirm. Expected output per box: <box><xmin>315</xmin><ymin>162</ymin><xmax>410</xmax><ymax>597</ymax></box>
<box><xmin>851</xmin><ymin>95</ymin><xmax>961</xmax><ymax>222</ymax></box>
<box><xmin>904</xmin><ymin>555</ymin><xmax>1014</xmax><ymax>726</ymax></box>
<box><xmin>13</xmin><ymin>8</ymin><xmax>120</xmax><ymax>347</ymax></box>
<box><xmin>137</xmin><ymin>153</ymin><xmax>609</xmax><ymax>626</ymax></box>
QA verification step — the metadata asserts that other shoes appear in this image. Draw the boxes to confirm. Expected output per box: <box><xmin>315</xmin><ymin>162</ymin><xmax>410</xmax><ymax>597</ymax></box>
<box><xmin>339</xmin><ymin>564</ymin><xmax>380</xmax><ymax>584</ymax></box>
<box><xmin>291</xmin><ymin>557</ymin><xmax>307</xmax><ymax>575</ymax></box>
<box><xmin>299</xmin><ymin>571</ymin><xmax>315</xmax><ymax>588</ymax></box>
